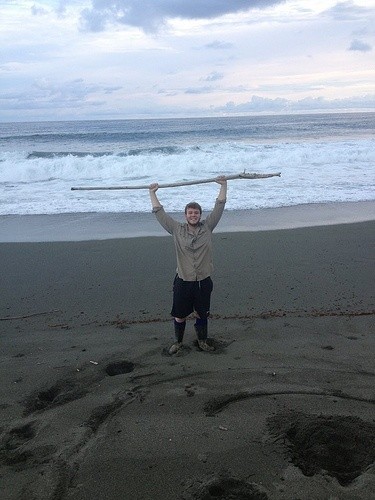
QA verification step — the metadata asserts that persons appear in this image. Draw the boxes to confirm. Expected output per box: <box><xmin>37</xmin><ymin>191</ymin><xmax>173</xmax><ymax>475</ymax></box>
<box><xmin>148</xmin><ymin>173</ymin><xmax>227</xmax><ymax>355</ymax></box>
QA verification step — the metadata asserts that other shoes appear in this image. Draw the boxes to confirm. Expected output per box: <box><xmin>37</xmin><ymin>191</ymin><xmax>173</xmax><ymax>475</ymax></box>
<box><xmin>169</xmin><ymin>343</ymin><xmax>184</xmax><ymax>355</ymax></box>
<box><xmin>198</xmin><ymin>339</ymin><xmax>215</xmax><ymax>352</ymax></box>
